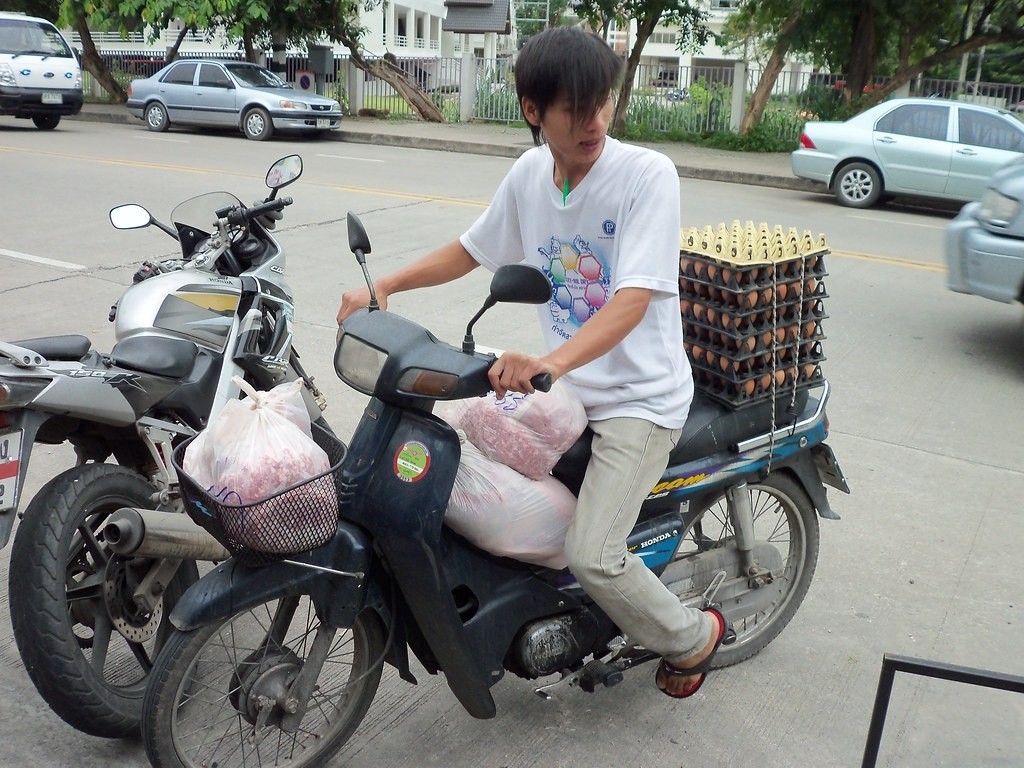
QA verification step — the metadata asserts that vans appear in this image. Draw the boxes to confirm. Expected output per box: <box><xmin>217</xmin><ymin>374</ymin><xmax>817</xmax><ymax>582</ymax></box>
<box><xmin>0</xmin><ymin>10</ymin><xmax>84</xmax><ymax>131</ymax></box>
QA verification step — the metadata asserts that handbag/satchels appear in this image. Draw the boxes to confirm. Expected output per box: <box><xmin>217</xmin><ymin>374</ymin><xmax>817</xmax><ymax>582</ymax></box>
<box><xmin>433</xmin><ymin>422</ymin><xmax>579</xmax><ymax>571</ymax></box>
<box><xmin>460</xmin><ymin>354</ymin><xmax>589</xmax><ymax>481</ymax></box>
<box><xmin>183</xmin><ymin>376</ymin><xmax>339</xmax><ymax>554</ymax></box>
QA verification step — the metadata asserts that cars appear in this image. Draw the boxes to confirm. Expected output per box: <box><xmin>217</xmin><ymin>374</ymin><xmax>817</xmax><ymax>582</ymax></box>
<box><xmin>652</xmin><ymin>70</ymin><xmax>678</xmax><ymax>88</ymax></box>
<box><xmin>943</xmin><ymin>152</ymin><xmax>1024</xmax><ymax>307</ymax></box>
<box><xmin>125</xmin><ymin>58</ymin><xmax>344</xmax><ymax>141</ymax></box>
<box><xmin>790</xmin><ymin>96</ymin><xmax>1024</xmax><ymax>210</ymax></box>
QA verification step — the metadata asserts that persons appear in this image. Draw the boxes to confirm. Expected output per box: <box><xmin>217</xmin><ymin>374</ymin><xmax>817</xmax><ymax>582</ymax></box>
<box><xmin>336</xmin><ymin>28</ymin><xmax>727</xmax><ymax>699</ymax></box>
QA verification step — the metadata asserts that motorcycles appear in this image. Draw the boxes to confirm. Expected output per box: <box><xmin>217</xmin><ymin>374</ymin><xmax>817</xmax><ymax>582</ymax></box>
<box><xmin>0</xmin><ymin>152</ymin><xmax>348</xmax><ymax>739</ymax></box>
<box><xmin>140</xmin><ymin>211</ymin><xmax>852</xmax><ymax>767</ymax></box>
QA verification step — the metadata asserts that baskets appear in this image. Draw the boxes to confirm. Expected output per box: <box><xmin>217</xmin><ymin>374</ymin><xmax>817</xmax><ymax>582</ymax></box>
<box><xmin>170</xmin><ymin>422</ymin><xmax>347</xmax><ymax>569</ymax></box>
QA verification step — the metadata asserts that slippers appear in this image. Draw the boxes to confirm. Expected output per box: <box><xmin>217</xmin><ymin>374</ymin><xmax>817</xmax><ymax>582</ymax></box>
<box><xmin>656</xmin><ymin>607</ymin><xmax>728</xmax><ymax>698</ymax></box>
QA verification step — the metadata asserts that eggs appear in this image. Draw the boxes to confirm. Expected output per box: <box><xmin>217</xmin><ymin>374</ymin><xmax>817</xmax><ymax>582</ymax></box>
<box><xmin>679</xmin><ymin>255</ymin><xmax>816</xmax><ymax>394</ymax></box>
